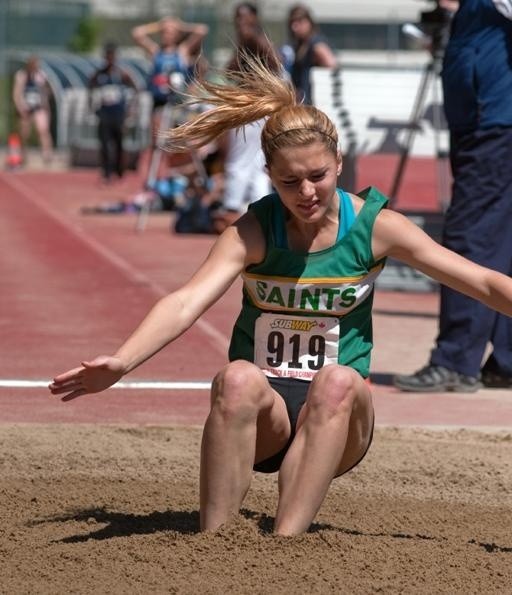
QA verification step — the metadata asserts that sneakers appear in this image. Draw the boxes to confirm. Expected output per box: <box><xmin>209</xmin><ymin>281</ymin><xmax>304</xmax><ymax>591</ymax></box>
<box><xmin>393</xmin><ymin>366</ymin><xmax>483</xmax><ymax>394</ymax></box>
<box><xmin>482</xmin><ymin>355</ymin><xmax>512</xmax><ymax>388</ymax></box>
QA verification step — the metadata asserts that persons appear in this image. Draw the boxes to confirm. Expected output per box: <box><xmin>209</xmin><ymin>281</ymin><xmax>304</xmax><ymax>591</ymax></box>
<box><xmin>132</xmin><ymin>15</ymin><xmax>210</xmax><ymax>187</ymax></box>
<box><xmin>286</xmin><ymin>7</ymin><xmax>337</xmax><ymax>107</ymax></box>
<box><xmin>13</xmin><ymin>52</ymin><xmax>58</xmax><ymax>162</ymax></box>
<box><xmin>390</xmin><ymin>1</ymin><xmax>512</xmax><ymax>393</ymax></box>
<box><xmin>215</xmin><ymin>3</ymin><xmax>279</xmax><ymax>237</ymax></box>
<box><xmin>49</xmin><ymin>40</ymin><xmax>512</xmax><ymax>539</ymax></box>
<box><xmin>87</xmin><ymin>40</ymin><xmax>140</xmax><ymax>182</ymax></box>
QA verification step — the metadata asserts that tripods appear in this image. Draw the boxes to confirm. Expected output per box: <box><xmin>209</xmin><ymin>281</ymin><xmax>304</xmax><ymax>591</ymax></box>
<box><xmin>386</xmin><ymin>54</ymin><xmax>452</xmax><ymax>213</ymax></box>
<box><xmin>132</xmin><ymin>99</ymin><xmax>209</xmax><ymax>235</ymax></box>
